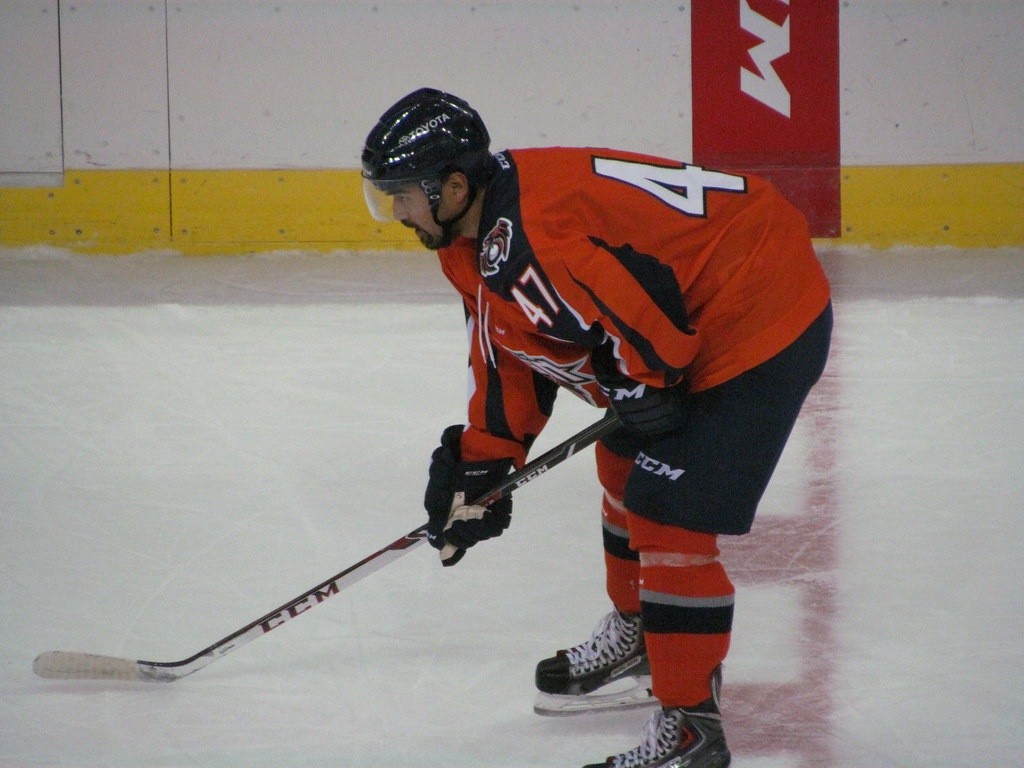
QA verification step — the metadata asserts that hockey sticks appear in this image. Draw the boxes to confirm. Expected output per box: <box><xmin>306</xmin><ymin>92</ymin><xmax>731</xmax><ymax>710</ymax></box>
<box><xmin>29</xmin><ymin>416</ymin><xmax>628</xmax><ymax>685</ymax></box>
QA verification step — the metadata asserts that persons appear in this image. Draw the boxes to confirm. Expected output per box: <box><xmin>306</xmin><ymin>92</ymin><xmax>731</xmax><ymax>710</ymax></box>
<box><xmin>359</xmin><ymin>88</ymin><xmax>833</xmax><ymax>768</ymax></box>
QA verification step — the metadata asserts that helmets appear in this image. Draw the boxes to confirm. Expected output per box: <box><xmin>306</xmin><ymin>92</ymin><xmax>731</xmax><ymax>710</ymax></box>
<box><xmin>362</xmin><ymin>88</ymin><xmax>491</xmax><ymax>192</ymax></box>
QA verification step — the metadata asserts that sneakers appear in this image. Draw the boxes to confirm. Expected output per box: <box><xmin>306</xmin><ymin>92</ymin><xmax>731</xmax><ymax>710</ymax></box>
<box><xmin>582</xmin><ymin>663</ymin><xmax>731</xmax><ymax>768</ymax></box>
<box><xmin>533</xmin><ymin>604</ymin><xmax>658</xmax><ymax>717</ymax></box>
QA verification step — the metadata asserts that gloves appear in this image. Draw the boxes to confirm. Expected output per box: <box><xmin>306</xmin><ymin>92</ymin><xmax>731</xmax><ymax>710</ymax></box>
<box><xmin>425</xmin><ymin>424</ymin><xmax>513</xmax><ymax>568</ymax></box>
<box><xmin>591</xmin><ymin>344</ymin><xmax>692</xmax><ymax>440</ymax></box>
